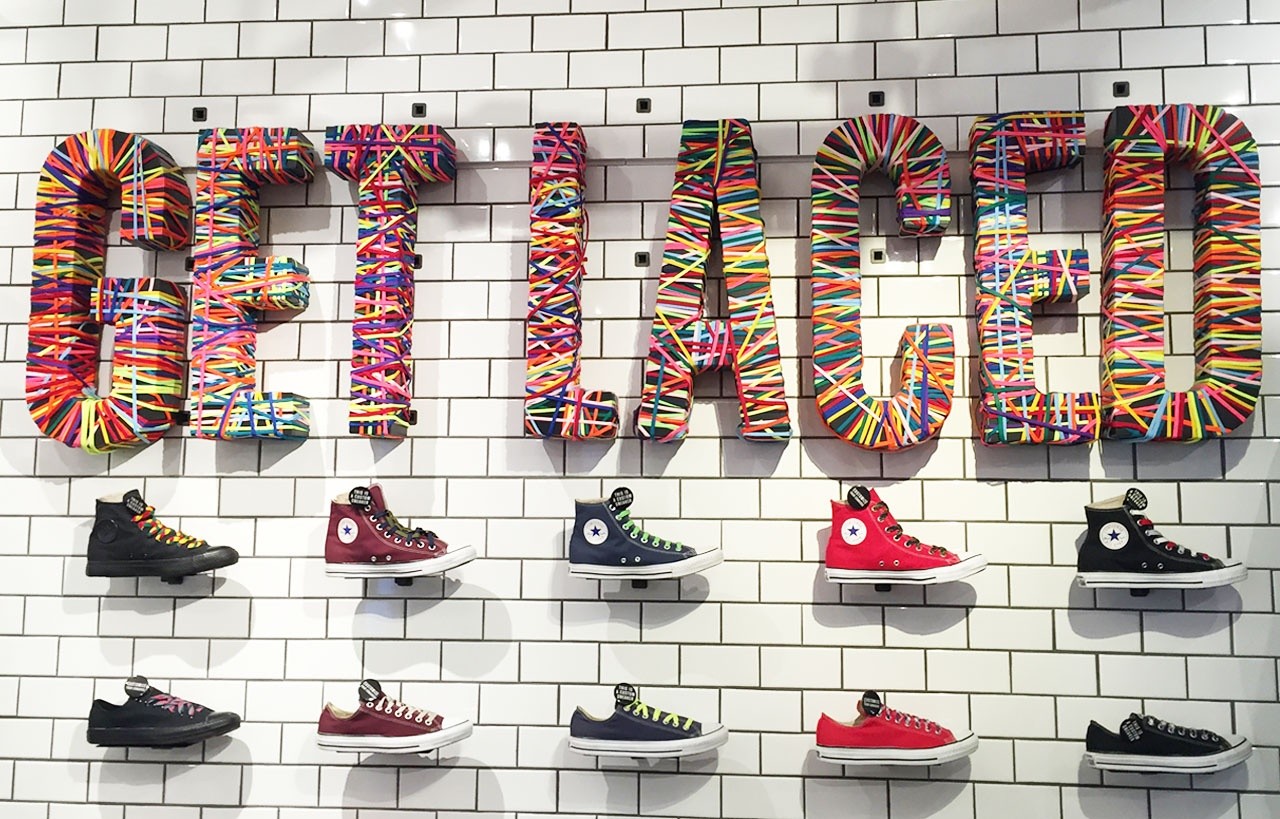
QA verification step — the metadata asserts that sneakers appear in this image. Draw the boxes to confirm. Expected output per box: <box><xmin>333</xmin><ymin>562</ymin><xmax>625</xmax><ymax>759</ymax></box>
<box><xmin>816</xmin><ymin>699</ymin><xmax>978</xmax><ymax>766</ymax></box>
<box><xmin>1074</xmin><ymin>495</ymin><xmax>1249</xmax><ymax>590</ymax></box>
<box><xmin>825</xmin><ymin>489</ymin><xmax>987</xmax><ymax>584</ymax></box>
<box><xmin>85</xmin><ymin>489</ymin><xmax>239</xmax><ymax>577</ymax></box>
<box><xmin>87</xmin><ymin>687</ymin><xmax>240</xmax><ymax>746</ymax></box>
<box><xmin>325</xmin><ymin>483</ymin><xmax>476</xmax><ymax>579</ymax></box>
<box><xmin>569</xmin><ymin>700</ymin><xmax>727</xmax><ymax>759</ymax></box>
<box><xmin>318</xmin><ymin>693</ymin><xmax>472</xmax><ymax>754</ymax></box>
<box><xmin>1086</xmin><ymin>714</ymin><xmax>1252</xmax><ymax>773</ymax></box>
<box><xmin>568</xmin><ymin>498</ymin><xmax>723</xmax><ymax>579</ymax></box>
